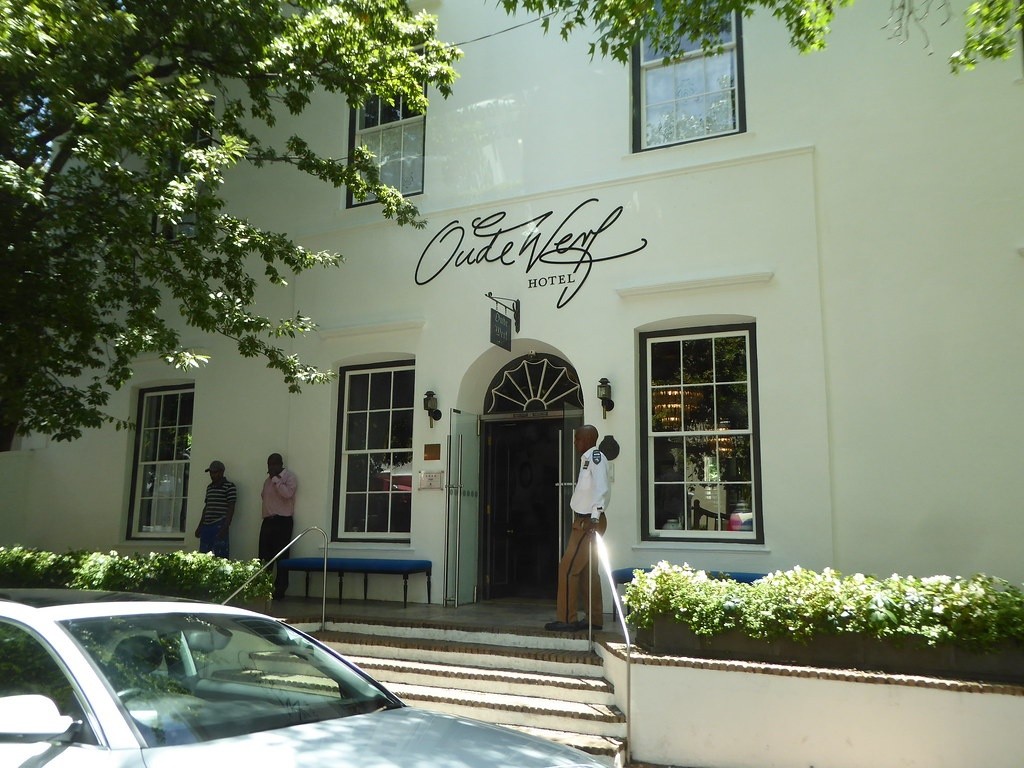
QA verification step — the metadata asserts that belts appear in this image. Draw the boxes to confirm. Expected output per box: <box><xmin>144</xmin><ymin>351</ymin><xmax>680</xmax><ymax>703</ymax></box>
<box><xmin>575</xmin><ymin>511</ymin><xmax>604</xmax><ymax>518</ymax></box>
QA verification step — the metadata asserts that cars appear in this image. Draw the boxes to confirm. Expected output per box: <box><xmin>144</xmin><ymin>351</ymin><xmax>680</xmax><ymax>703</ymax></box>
<box><xmin>1</xmin><ymin>585</ymin><xmax>618</xmax><ymax>768</ymax></box>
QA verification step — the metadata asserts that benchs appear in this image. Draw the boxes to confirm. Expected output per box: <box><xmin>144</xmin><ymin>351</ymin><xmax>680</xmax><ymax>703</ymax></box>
<box><xmin>274</xmin><ymin>555</ymin><xmax>434</xmax><ymax>608</ymax></box>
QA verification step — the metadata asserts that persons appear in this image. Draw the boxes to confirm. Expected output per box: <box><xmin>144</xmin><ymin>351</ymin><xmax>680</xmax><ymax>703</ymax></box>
<box><xmin>258</xmin><ymin>453</ymin><xmax>297</xmax><ymax>601</ymax></box>
<box><xmin>544</xmin><ymin>425</ymin><xmax>610</xmax><ymax>631</ymax></box>
<box><xmin>195</xmin><ymin>461</ymin><xmax>237</xmax><ymax>559</ymax></box>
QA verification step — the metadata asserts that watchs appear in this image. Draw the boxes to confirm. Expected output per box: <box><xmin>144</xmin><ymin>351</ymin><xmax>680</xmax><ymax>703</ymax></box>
<box><xmin>591</xmin><ymin>518</ymin><xmax>599</xmax><ymax>524</ymax></box>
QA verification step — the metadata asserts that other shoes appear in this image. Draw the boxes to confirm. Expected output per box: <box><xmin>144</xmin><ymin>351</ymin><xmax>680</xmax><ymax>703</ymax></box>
<box><xmin>271</xmin><ymin>589</ymin><xmax>285</xmax><ymax>599</ymax></box>
<box><xmin>578</xmin><ymin>618</ymin><xmax>602</xmax><ymax>630</ymax></box>
<box><xmin>545</xmin><ymin>621</ymin><xmax>578</xmax><ymax>632</ymax></box>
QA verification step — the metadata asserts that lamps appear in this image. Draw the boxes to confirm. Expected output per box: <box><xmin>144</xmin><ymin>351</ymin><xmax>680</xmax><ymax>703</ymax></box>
<box><xmin>423</xmin><ymin>390</ymin><xmax>441</xmax><ymax>429</ymax></box>
<box><xmin>597</xmin><ymin>378</ymin><xmax>613</xmax><ymax>419</ymax></box>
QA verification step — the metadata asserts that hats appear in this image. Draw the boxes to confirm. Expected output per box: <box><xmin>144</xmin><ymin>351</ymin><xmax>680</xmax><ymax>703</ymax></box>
<box><xmin>205</xmin><ymin>460</ymin><xmax>225</xmax><ymax>473</ymax></box>
